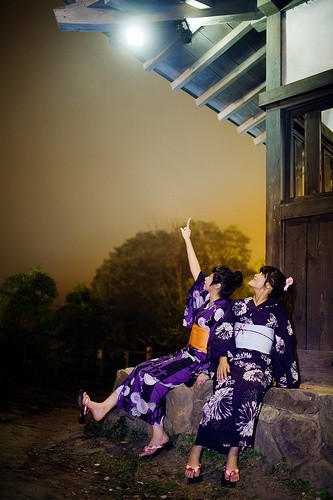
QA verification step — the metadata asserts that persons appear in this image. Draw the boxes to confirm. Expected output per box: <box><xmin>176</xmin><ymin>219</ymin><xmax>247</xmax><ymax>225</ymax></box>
<box><xmin>185</xmin><ymin>266</ymin><xmax>302</xmax><ymax>486</ymax></box>
<box><xmin>81</xmin><ymin>218</ymin><xmax>244</xmax><ymax>461</ymax></box>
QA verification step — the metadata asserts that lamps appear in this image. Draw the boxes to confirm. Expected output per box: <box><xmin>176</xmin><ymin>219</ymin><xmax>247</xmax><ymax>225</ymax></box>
<box><xmin>175</xmin><ymin>19</ymin><xmax>192</xmax><ymax>44</ymax></box>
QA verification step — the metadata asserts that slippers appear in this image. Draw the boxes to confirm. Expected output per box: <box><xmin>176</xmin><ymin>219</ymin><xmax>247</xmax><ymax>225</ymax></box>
<box><xmin>78</xmin><ymin>389</ymin><xmax>90</xmax><ymax>424</ymax></box>
<box><xmin>184</xmin><ymin>452</ymin><xmax>202</xmax><ymax>484</ymax></box>
<box><xmin>138</xmin><ymin>438</ymin><xmax>172</xmax><ymax>459</ymax></box>
<box><xmin>221</xmin><ymin>465</ymin><xmax>240</xmax><ymax>488</ymax></box>
